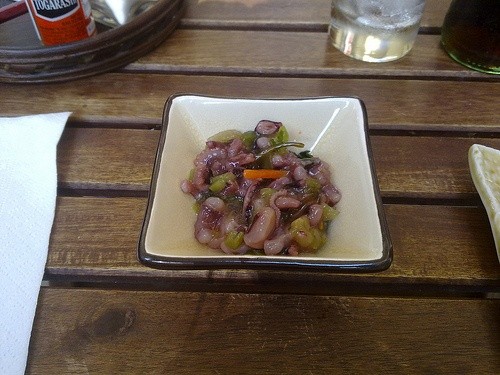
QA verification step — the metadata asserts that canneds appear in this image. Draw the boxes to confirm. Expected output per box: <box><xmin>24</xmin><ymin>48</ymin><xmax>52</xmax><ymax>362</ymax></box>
<box><xmin>25</xmin><ymin>0</ymin><xmax>98</xmax><ymax>45</ymax></box>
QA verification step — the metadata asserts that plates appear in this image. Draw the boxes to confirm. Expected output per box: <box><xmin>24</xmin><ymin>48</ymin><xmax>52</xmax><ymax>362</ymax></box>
<box><xmin>137</xmin><ymin>92</ymin><xmax>394</xmax><ymax>274</ymax></box>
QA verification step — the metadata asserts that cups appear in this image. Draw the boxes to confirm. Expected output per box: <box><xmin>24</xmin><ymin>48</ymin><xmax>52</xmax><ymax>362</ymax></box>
<box><xmin>328</xmin><ymin>0</ymin><xmax>426</xmax><ymax>63</ymax></box>
<box><xmin>440</xmin><ymin>0</ymin><xmax>500</xmax><ymax>75</ymax></box>
